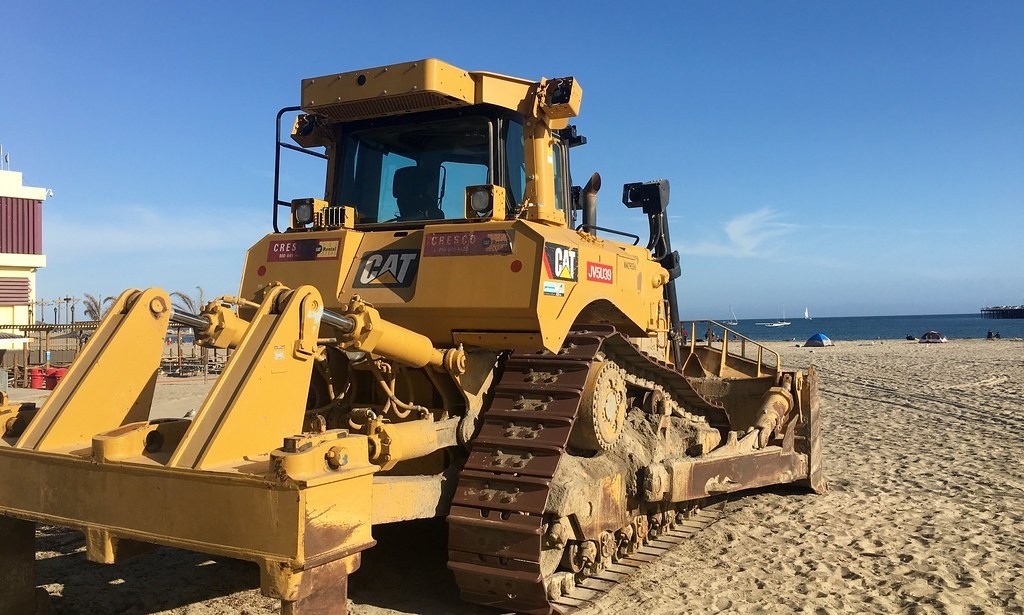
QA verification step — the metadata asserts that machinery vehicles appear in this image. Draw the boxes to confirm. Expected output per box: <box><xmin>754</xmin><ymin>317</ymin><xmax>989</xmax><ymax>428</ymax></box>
<box><xmin>0</xmin><ymin>57</ymin><xmax>829</xmax><ymax>615</ymax></box>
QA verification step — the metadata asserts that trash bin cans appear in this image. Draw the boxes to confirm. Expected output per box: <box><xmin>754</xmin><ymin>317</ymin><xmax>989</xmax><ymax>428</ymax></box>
<box><xmin>29</xmin><ymin>367</ymin><xmax>45</xmax><ymax>389</ymax></box>
<box><xmin>46</xmin><ymin>367</ymin><xmax>67</xmax><ymax>390</ymax></box>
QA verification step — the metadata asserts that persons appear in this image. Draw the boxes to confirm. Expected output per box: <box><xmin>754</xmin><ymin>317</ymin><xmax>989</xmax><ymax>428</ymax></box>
<box><xmin>705</xmin><ymin>330</ymin><xmax>725</xmax><ymax>342</ymax></box>
<box><xmin>987</xmin><ymin>330</ymin><xmax>994</xmax><ymax>339</ymax></box>
<box><xmin>906</xmin><ymin>333</ymin><xmax>919</xmax><ymax>341</ymax></box>
<box><xmin>993</xmin><ymin>331</ymin><xmax>1000</xmax><ymax>339</ymax></box>
<box><xmin>165</xmin><ymin>336</ymin><xmax>183</xmax><ymax>345</ymax></box>
<box><xmin>683</xmin><ymin>328</ymin><xmax>688</xmax><ymax>344</ymax></box>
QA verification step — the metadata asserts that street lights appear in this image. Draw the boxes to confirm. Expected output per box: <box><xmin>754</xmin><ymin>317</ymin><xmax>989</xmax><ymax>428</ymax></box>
<box><xmin>70</xmin><ymin>303</ymin><xmax>75</xmax><ymax>324</ymax></box>
<box><xmin>54</xmin><ymin>306</ymin><xmax>58</xmax><ymax>324</ymax></box>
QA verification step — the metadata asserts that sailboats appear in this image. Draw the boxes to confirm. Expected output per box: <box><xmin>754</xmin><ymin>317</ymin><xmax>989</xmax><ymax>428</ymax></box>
<box><xmin>754</xmin><ymin>303</ymin><xmax>792</xmax><ymax>327</ymax></box>
<box><xmin>804</xmin><ymin>307</ymin><xmax>813</xmax><ymax>321</ymax></box>
<box><xmin>721</xmin><ymin>305</ymin><xmax>738</xmax><ymax>326</ymax></box>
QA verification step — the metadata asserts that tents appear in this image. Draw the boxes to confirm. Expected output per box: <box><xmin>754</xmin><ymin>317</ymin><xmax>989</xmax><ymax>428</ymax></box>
<box><xmin>918</xmin><ymin>330</ymin><xmax>947</xmax><ymax>343</ymax></box>
<box><xmin>801</xmin><ymin>333</ymin><xmax>835</xmax><ymax>347</ymax></box>
